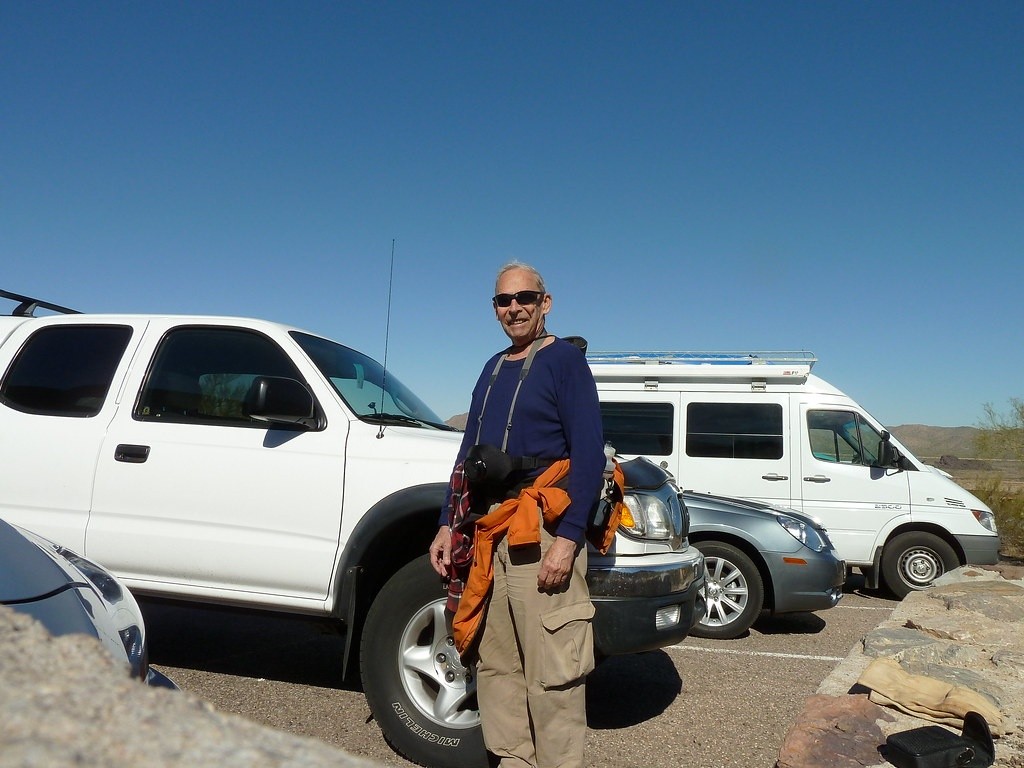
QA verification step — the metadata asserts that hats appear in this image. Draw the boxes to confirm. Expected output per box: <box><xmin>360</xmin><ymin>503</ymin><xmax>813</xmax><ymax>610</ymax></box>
<box><xmin>560</xmin><ymin>335</ymin><xmax>587</xmax><ymax>357</ymax></box>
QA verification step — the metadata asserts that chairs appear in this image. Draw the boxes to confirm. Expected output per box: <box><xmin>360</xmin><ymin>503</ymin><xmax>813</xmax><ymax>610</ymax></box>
<box><xmin>151</xmin><ymin>367</ymin><xmax>202</xmax><ymax>417</ymax></box>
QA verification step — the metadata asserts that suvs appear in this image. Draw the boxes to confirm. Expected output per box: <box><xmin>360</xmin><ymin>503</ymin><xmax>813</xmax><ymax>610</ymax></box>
<box><xmin>0</xmin><ymin>289</ymin><xmax>706</xmax><ymax>767</ymax></box>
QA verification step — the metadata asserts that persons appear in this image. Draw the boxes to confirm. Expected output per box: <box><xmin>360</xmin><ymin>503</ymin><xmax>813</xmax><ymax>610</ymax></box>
<box><xmin>429</xmin><ymin>262</ymin><xmax>607</xmax><ymax>768</ymax></box>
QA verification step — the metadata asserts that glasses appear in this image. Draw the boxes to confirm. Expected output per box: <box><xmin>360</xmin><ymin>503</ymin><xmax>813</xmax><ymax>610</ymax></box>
<box><xmin>491</xmin><ymin>290</ymin><xmax>545</xmax><ymax>307</ymax></box>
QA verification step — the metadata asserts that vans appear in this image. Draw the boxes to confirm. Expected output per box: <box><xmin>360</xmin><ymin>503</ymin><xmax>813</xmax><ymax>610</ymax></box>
<box><xmin>584</xmin><ymin>350</ymin><xmax>1001</xmax><ymax>597</ymax></box>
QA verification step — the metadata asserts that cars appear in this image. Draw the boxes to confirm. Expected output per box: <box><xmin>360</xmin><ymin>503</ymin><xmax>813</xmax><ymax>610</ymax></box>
<box><xmin>677</xmin><ymin>488</ymin><xmax>847</xmax><ymax>639</ymax></box>
<box><xmin>1</xmin><ymin>516</ymin><xmax>149</xmax><ymax>684</ymax></box>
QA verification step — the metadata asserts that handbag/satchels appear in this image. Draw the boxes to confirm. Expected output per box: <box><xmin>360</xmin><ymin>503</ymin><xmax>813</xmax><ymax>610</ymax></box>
<box><xmin>886</xmin><ymin>709</ymin><xmax>996</xmax><ymax>768</ymax></box>
